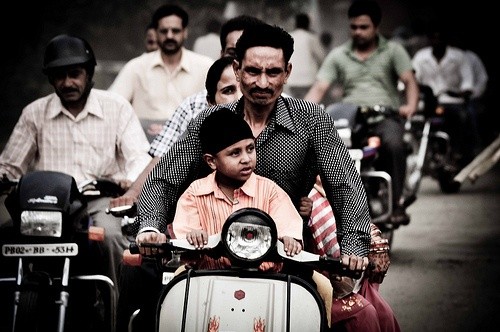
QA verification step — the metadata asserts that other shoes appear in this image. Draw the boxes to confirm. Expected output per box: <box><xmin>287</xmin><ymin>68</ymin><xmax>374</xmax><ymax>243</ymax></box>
<box><xmin>386</xmin><ymin>203</ymin><xmax>405</xmax><ymax>224</ymax></box>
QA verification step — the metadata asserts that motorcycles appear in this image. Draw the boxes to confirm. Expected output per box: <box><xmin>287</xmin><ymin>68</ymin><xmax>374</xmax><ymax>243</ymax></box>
<box><xmin>129</xmin><ymin>207</ymin><xmax>374</xmax><ymax>332</ymax></box>
<box><xmin>317</xmin><ymin>70</ymin><xmax>500</xmax><ymax>257</ymax></box>
<box><xmin>0</xmin><ymin>171</ymin><xmax>125</xmax><ymax>332</ymax></box>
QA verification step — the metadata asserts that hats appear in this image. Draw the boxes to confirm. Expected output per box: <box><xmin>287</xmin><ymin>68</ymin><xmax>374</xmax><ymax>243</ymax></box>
<box><xmin>198</xmin><ymin>107</ymin><xmax>255</xmax><ymax>158</ymax></box>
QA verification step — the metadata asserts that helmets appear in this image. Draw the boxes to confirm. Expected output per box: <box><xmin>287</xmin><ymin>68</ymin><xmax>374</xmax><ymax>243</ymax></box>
<box><xmin>40</xmin><ymin>34</ymin><xmax>97</xmax><ymax>83</ymax></box>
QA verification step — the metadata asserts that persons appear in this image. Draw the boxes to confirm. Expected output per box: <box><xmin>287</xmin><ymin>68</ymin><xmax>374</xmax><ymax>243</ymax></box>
<box><xmin>104</xmin><ymin>3</ymin><xmax>214</xmax><ymax>144</ymax></box>
<box><xmin>133</xmin><ymin>15</ymin><xmax>403</xmax><ymax>332</ymax></box>
<box><xmin>0</xmin><ymin>32</ymin><xmax>154</xmax><ymax>331</ymax></box>
<box><xmin>282</xmin><ymin>11</ymin><xmax>327</xmax><ymax>100</ymax></box>
<box><xmin>303</xmin><ymin>0</ymin><xmax>419</xmax><ymax>226</ymax></box>
<box><xmin>397</xmin><ymin>19</ymin><xmax>490</xmax><ymax>165</ymax></box>
